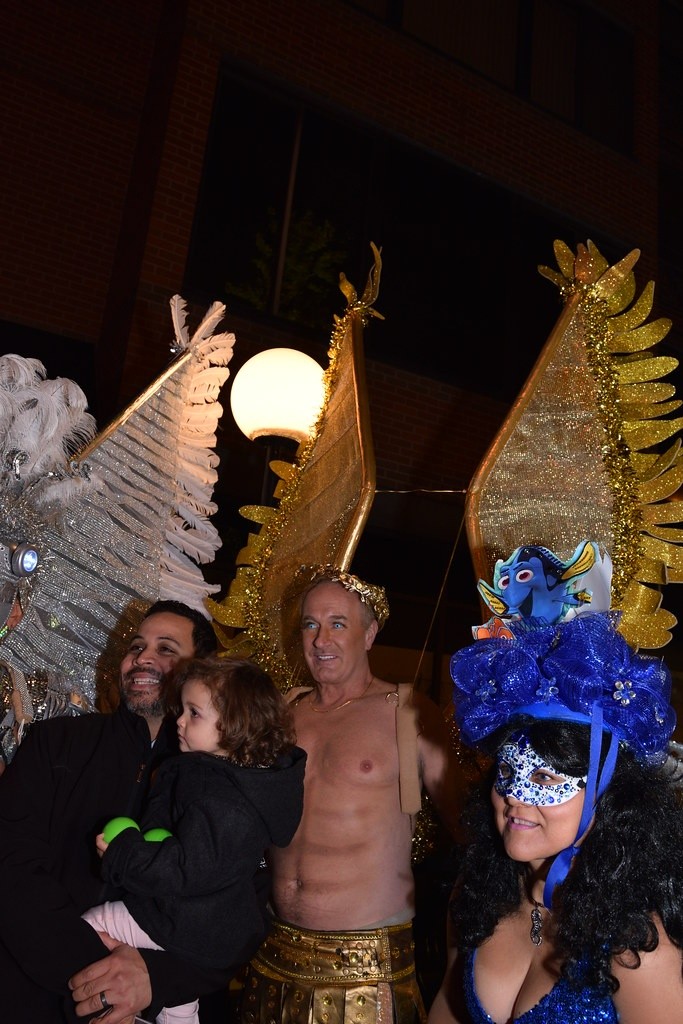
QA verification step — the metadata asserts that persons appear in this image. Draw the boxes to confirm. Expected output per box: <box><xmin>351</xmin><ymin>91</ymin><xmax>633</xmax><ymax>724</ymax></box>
<box><xmin>0</xmin><ymin>598</ymin><xmax>267</xmax><ymax>1024</ymax></box>
<box><xmin>0</xmin><ymin>541</ymin><xmax>97</xmax><ymax>775</ymax></box>
<box><xmin>426</xmin><ymin>716</ymin><xmax>683</xmax><ymax>1024</ymax></box>
<box><xmin>232</xmin><ymin>562</ymin><xmax>482</xmax><ymax>1024</ymax></box>
<box><xmin>61</xmin><ymin>655</ymin><xmax>307</xmax><ymax>1024</ymax></box>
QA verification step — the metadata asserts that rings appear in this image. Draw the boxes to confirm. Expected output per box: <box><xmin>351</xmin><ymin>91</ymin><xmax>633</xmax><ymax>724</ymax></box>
<box><xmin>100</xmin><ymin>992</ymin><xmax>108</xmax><ymax>1007</ymax></box>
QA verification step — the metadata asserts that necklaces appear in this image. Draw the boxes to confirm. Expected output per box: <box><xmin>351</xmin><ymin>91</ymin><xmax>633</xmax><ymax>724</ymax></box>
<box><xmin>527</xmin><ymin>895</ymin><xmax>547</xmax><ymax>946</ymax></box>
<box><xmin>309</xmin><ymin>675</ymin><xmax>375</xmax><ymax>713</ymax></box>
